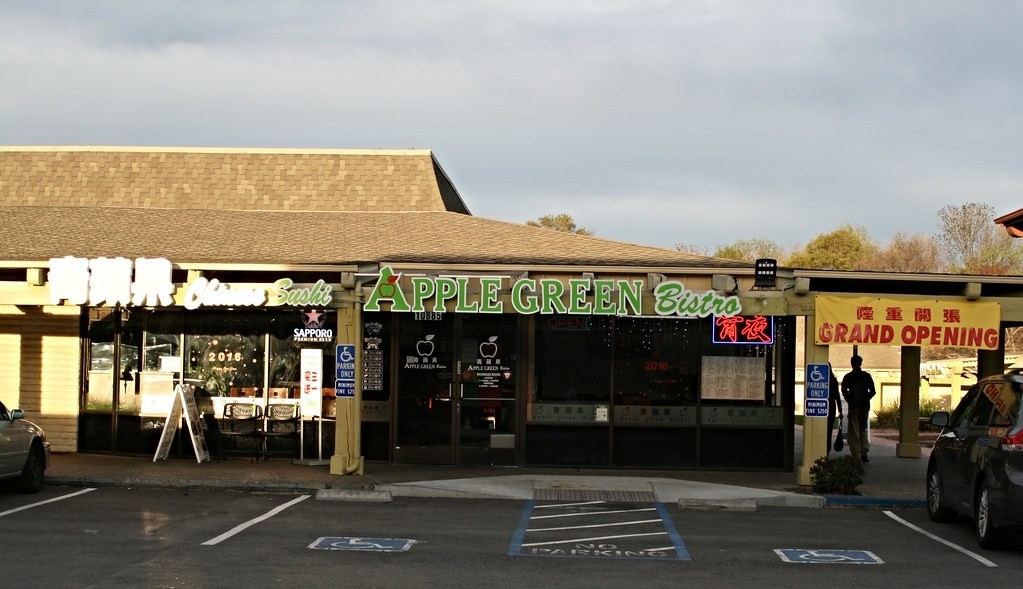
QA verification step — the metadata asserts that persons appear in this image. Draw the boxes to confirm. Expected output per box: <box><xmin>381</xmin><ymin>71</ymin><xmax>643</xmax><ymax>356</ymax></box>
<box><xmin>840</xmin><ymin>355</ymin><xmax>877</xmax><ymax>463</ymax></box>
<box><xmin>827</xmin><ymin>360</ymin><xmax>843</xmax><ymax>457</ymax></box>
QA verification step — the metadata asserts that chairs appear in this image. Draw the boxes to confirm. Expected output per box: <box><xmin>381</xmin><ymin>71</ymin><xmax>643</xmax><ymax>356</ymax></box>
<box><xmin>215</xmin><ymin>403</ymin><xmax>262</xmax><ymax>464</ymax></box>
<box><xmin>258</xmin><ymin>404</ymin><xmax>301</xmax><ymax>464</ymax></box>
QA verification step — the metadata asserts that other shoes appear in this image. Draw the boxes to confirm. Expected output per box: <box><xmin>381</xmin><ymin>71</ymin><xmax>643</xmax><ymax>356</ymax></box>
<box><xmin>861</xmin><ymin>448</ymin><xmax>869</xmax><ymax>463</ymax></box>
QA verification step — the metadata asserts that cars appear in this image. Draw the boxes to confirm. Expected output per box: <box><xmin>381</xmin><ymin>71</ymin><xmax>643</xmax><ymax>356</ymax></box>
<box><xmin>0</xmin><ymin>402</ymin><xmax>50</xmax><ymax>494</ymax></box>
<box><xmin>925</xmin><ymin>366</ymin><xmax>1023</xmax><ymax>550</ymax></box>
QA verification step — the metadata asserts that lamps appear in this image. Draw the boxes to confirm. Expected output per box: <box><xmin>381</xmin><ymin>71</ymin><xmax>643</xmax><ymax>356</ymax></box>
<box><xmin>755</xmin><ymin>259</ymin><xmax>777</xmax><ymax>287</ymax></box>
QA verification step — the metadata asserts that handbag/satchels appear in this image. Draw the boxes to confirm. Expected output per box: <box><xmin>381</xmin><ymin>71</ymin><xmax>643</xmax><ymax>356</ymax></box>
<box><xmin>834</xmin><ymin>419</ymin><xmax>844</xmax><ymax>452</ymax></box>
